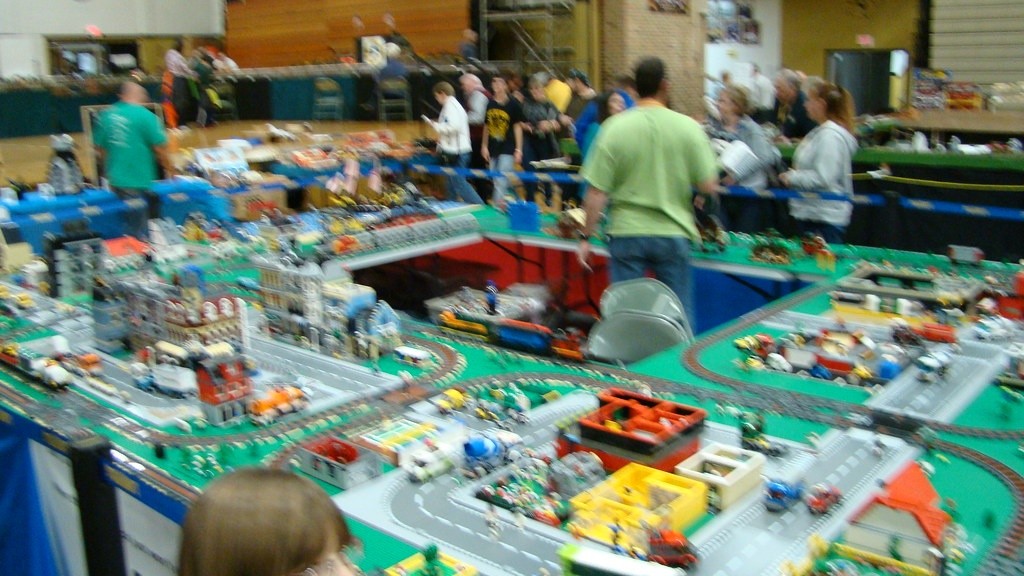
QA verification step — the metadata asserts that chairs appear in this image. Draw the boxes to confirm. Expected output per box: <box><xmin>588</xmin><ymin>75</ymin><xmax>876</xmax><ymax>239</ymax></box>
<box><xmin>312</xmin><ymin>76</ymin><xmax>351</xmax><ymax>127</ymax></box>
<box><xmin>375</xmin><ymin>76</ymin><xmax>412</xmax><ymax>125</ymax></box>
<box><xmin>584</xmin><ymin>309</ymin><xmax>688</xmax><ymax>366</ymax></box>
<box><xmin>599</xmin><ymin>279</ymin><xmax>693</xmax><ymax>343</ymax></box>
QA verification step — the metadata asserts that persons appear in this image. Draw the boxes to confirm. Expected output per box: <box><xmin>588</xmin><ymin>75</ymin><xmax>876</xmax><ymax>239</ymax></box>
<box><xmin>461</xmin><ymin>30</ymin><xmax>482</xmax><ymax>65</ymax></box>
<box><xmin>373</xmin><ymin>43</ymin><xmax>407</xmax><ymax>111</ymax></box>
<box><xmin>166</xmin><ymin>40</ymin><xmax>239</xmax><ymax>131</ymax></box>
<box><xmin>774</xmin><ymin>69</ymin><xmax>817</xmax><ymax>139</ymax></box>
<box><xmin>177</xmin><ymin>469</ymin><xmax>360</xmax><ymax>576</ymax></box>
<box><xmin>94</xmin><ymin>82</ymin><xmax>168</xmax><ymax>197</ymax></box>
<box><xmin>575</xmin><ymin>77</ymin><xmax>636</xmax><ymax>152</ymax></box>
<box><xmin>779</xmin><ymin>83</ymin><xmax>858</xmax><ymax>244</ymax></box>
<box><xmin>577</xmin><ymin>54</ymin><xmax>719</xmax><ymax>336</ymax></box>
<box><xmin>422</xmin><ymin>68</ymin><xmax>597</xmax><ymax>206</ymax></box>
<box><xmin>46</xmin><ymin>134</ymin><xmax>85</xmax><ymax>195</ymax></box>
<box><xmin>714</xmin><ymin>86</ymin><xmax>775</xmax><ymax>234</ymax></box>
<box><xmin>581</xmin><ymin>91</ymin><xmax>627</xmax><ymax>201</ymax></box>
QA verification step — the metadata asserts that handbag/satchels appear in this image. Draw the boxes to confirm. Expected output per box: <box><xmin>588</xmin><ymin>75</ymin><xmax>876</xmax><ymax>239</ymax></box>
<box><xmin>438</xmin><ymin>151</ymin><xmax>459</xmax><ymax>166</ymax></box>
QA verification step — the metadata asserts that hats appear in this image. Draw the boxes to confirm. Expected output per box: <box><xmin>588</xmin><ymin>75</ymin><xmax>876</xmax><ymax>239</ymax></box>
<box><xmin>640</xmin><ymin>57</ymin><xmax>663</xmax><ymax>75</ymax></box>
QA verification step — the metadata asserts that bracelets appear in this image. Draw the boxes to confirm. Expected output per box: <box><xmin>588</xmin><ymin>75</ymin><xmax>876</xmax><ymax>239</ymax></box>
<box><xmin>515</xmin><ymin>148</ymin><xmax>521</xmax><ymax>154</ymax></box>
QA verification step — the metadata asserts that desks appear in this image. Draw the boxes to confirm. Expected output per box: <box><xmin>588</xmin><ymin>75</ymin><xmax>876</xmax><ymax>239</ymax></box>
<box><xmin>0</xmin><ymin>207</ymin><xmax>1024</xmax><ymax>576</ymax></box>
<box><xmin>562</xmin><ymin>115</ymin><xmax>1024</xmax><ymax>263</ymax></box>
<box><xmin>0</xmin><ymin>58</ymin><xmax>500</xmax><ymax>140</ymax></box>
